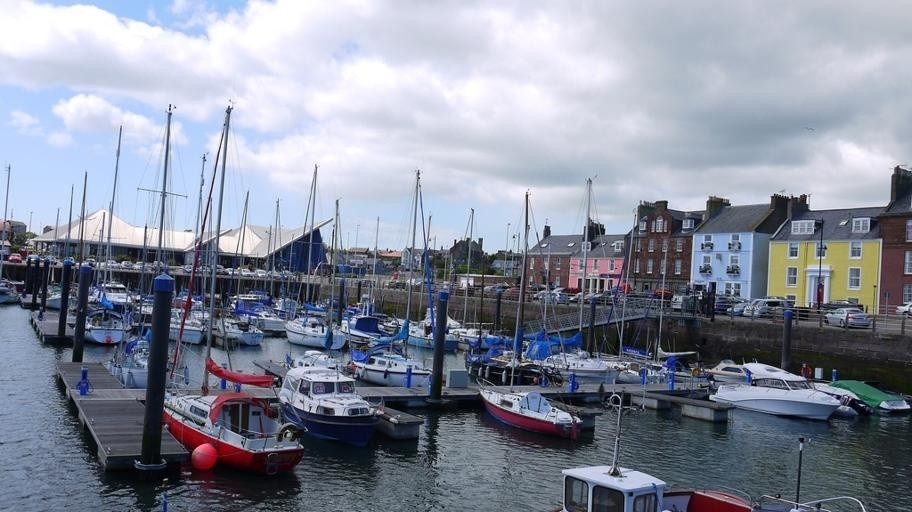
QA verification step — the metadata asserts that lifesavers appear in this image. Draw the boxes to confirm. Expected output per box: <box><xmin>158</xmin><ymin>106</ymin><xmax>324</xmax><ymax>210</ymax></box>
<box><xmin>801</xmin><ymin>364</ymin><xmax>811</xmax><ymax>377</ymax></box>
<box><xmin>693</xmin><ymin>368</ymin><xmax>700</xmax><ymax>376</ymax></box>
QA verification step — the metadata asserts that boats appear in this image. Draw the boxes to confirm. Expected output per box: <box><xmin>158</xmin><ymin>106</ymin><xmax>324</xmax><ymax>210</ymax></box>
<box><xmin>549</xmin><ymin>389</ymin><xmax>866</xmax><ymax>512</ymax></box>
